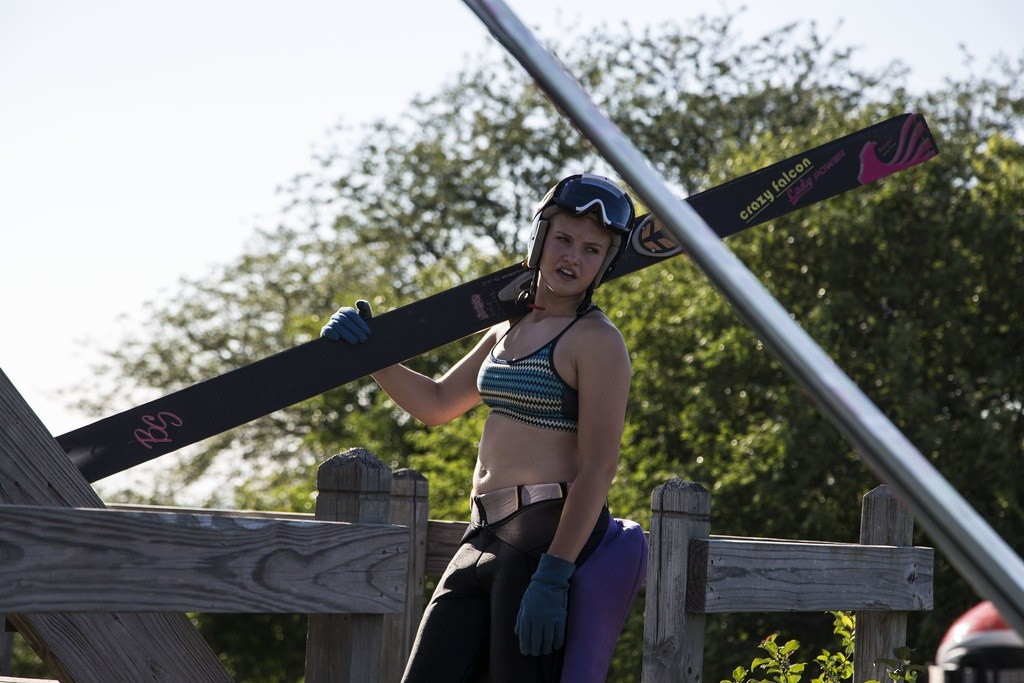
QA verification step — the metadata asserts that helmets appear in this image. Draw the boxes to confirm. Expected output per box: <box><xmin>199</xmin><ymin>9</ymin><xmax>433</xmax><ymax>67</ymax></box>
<box><xmin>527</xmin><ymin>181</ymin><xmax>634</xmax><ymax>290</ymax></box>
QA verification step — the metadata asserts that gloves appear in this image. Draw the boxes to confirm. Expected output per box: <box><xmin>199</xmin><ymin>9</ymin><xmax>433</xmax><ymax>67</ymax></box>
<box><xmin>514</xmin><ymin>553</ymin><xmax>576</xmax><ymax>656</ymax></box>
<box><xmin>320</xmin><ymin>299</ymin><xmax>373</xmax><ymax>344</ymax></box>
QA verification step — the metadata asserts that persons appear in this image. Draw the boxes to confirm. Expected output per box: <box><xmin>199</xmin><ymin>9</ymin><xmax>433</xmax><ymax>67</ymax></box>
<box><xmin>320</xmin><ymin>174</ymin><xmax>632</xmax><ymax>683</ymax></box>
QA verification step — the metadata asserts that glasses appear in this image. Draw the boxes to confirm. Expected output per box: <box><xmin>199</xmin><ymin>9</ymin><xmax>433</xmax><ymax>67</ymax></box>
<box><xmin>552</xmin><ymin>173</ymin><xmax>635</xmax><ymax>233</ymax></box>
